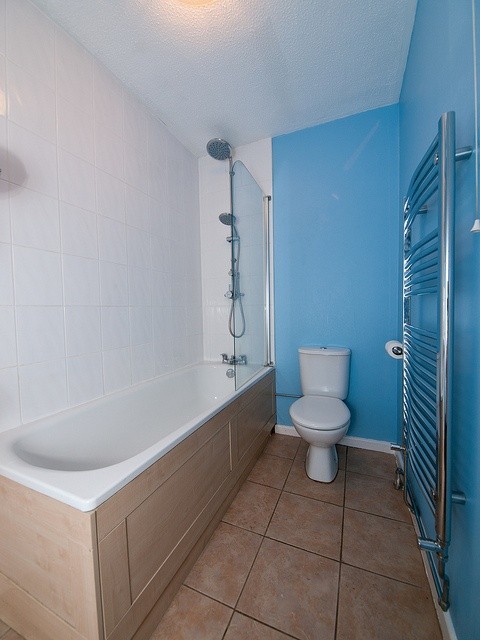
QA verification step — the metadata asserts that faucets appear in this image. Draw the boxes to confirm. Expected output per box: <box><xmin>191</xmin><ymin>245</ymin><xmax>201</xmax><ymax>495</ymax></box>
<box><xmin>230</xmin><ymin>354</ymin><xmax>237</xmax><ymax>365</ymax></box>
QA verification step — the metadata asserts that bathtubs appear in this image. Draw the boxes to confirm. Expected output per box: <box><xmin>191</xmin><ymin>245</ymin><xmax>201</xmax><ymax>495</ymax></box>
<box><xmin>0</xmin><ymin>360</ymin><xmax>275</xmax><ymax>634</ymax></box>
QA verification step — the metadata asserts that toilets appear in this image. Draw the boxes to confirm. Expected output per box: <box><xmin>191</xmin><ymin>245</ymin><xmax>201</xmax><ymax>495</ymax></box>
<box><xmin>289</xmin><ymin>346</ymin><xmax>351</xmax><ymax>483</ymax></box>
